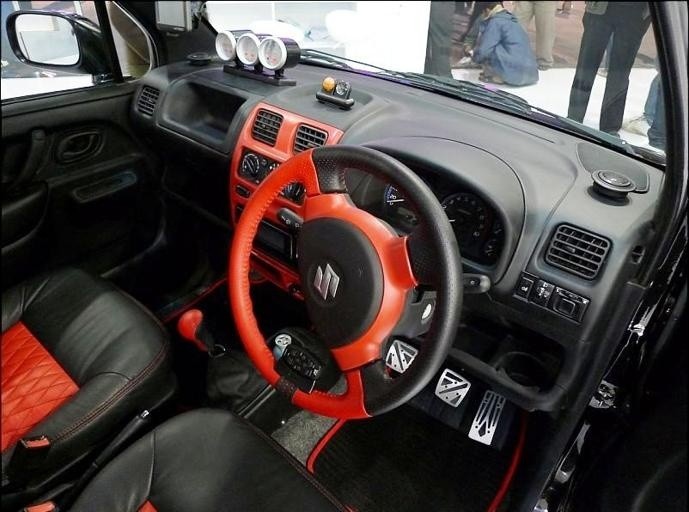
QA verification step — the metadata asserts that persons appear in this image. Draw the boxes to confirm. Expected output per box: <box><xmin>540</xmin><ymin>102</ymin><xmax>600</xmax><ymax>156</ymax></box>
<box><xmin>453</xmin><ymin>1</ymin><xmax>475</xmax><ymax>18</ymax></box>
<box><xmin>462</xmin><ymin>0</ymin><xmax>541</xmax><ymax>88</ymax></box>
<box><xmin>567</xmin><ymin>1</ymin><xmax>652</xmax><ymax>140</ymax></box>
<box><xmin>509</xmin><ymin>0</ymin><xmax>574</xmax><ymax>71</ymax></box>
<box><xmin>451</xmin><ymin>0</ymin><xmax>505</xmax><ymax>46</ymax></box>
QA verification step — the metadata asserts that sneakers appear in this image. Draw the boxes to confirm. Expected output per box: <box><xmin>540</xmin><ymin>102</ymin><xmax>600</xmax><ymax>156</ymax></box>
<box><xmin>622</xmin><ymin>114</ymin><xmax>650</xmax><ymax>137</ymax></box>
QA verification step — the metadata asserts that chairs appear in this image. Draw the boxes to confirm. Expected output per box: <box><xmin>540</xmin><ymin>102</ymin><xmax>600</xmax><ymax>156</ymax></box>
<box><xmin>70</xmin><ymin>408</ymin><xmax>343</xmax><ymax>512</ymax></box>
<box><xmin>0</xmin><ymin>265</ymin><xmax>178</xmax><ymax>504</ymax></box>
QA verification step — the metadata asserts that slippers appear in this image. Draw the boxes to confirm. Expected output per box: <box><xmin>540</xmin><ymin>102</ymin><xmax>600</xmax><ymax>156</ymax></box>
<box><xmin>479</xmin><ymin>72</ymin><xmax>505</xmax><ymax>85</ymax></box>
